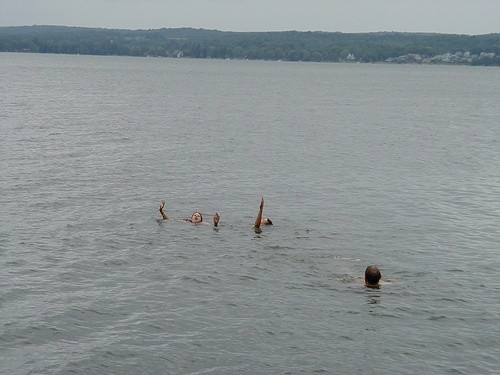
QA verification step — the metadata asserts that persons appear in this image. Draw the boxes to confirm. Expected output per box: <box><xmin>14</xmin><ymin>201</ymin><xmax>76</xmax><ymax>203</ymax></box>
<box><xmin>158</xmin><ymin>200</ymin><xmax>220</xmax><ymax>227</ymax></box>
<box><xmin>364</xmin><ymin>265</ymin><xmax>398</xmax><ymax>285</ymax></box>
<box><xmin>254</xmin><ymin>196</ymin><xmax>273</xmax><ymax>228</ymax></box>
